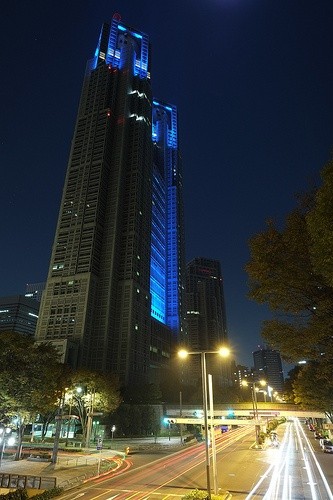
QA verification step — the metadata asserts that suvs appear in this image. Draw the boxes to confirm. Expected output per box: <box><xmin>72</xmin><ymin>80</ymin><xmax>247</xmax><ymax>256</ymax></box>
<box><xmin>304</xmin><ymin>418</ymin><xmax>333</xmax><ymax>454</ymax></box>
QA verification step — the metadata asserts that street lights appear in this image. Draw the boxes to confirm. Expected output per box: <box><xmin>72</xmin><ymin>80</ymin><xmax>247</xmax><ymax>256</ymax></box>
<box><xmin>176</xmin><ymin>346</ymin><xmax>230</xmax><ymax>500</ymax></box>
<box><xmin>242</xmin><ymin>380</ymin><xmax>285</xmax><ymax>438</ymax></box>
